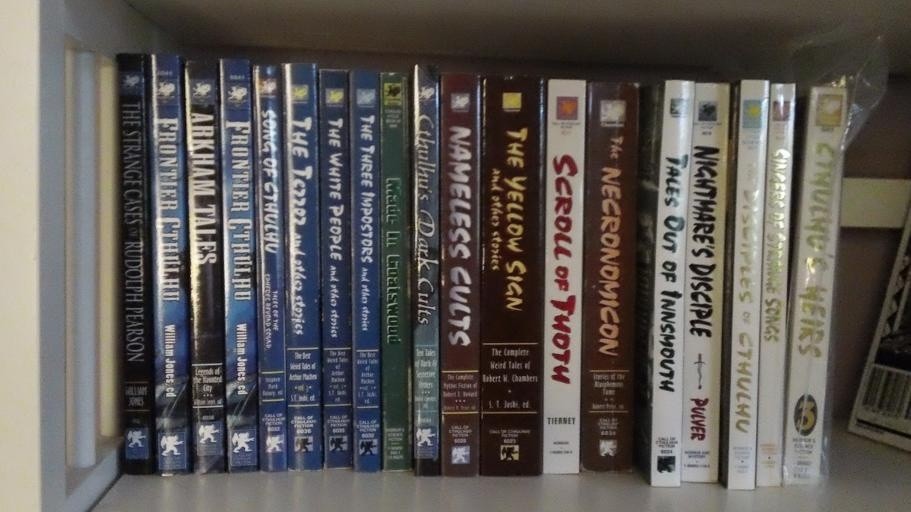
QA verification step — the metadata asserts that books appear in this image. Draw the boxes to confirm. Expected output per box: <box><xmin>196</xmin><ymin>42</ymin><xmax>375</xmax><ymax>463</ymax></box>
<box><xmin>319</xmin><ymin>69</ymin><xmax>349</xmax><ymax>468</ymax></box>
<box><xmin>148</xmin><ymin>53</ymin><xmax>192</xmax><ymax>475</ymax></box>
<box><xmin>349</xmin><ymin>68</ymin><xmax>381</xmax><ymax>472</ymax></box>
<box><xmin>539</xmin><ymin>79</ymin><xmax>587</xmax><ymax>474</ymax></box>
<box><xmin>442</xmin><ymin>74</ymin><xmax>483</xmax><ymax>479</ymax></box>
<box><xmin>184</xmin><ymin>58</ymin><xmax>227</xmax><ymax>472</ymax></box>
<box><xmin>849</xmin><ymin>184</ymin><xmax>910</xmax><ymax>451</ymax></box>
<box><xmin>414</xmin><ymin>64</ymin><xmax>443</xmax><ymax>474</ymax></box>
<box><xmin>116</xmin><ymin>52</ymin><xmax>153</xmax><ymax>474</ymax></box>
<box><xmin>632</xmin><ymin>79</ymin><xmax>696</xmax><ymax>488</ymax></box>
<box><xmin>581</xmin><ymin>81</ymin><xmax>642</xmax><ymax>475</ymax></box>
<box><xmin>253</xmin><ymin>64</ymin><xmax>288</xmax><ymax>472</ymax></box>
<box><xmin>681</xmin><ymin>83</ymin><xmax>730</xmax><ymax>484</ymax></box>
<box><xmin>219</xmin><ymin>58</ymin><xmax>259</xmax><ymax>473</ymax></box>
<box><xmin>283</xmin><ymin>63</ymin><xmax>319</xmax><ymax>470</ymax></box>
<box><xmin>719</xmin><ymin>80</ymin><xmax>769</xmax><ymax>489</ymax></box>
<box><xmin>754</xmin><ymin>84</ymin><xmax>799</xmax><ymax>487</ymax></box>
<box><xmin>380</xmin><ymin>71</ymin><xmax>413</xmax><ymax>472</ymax></box>
<box><xmin>784</xmin><ymin>88</ymin><xmax>845</xmax><ymax>484</ymax></box>
<box><xmin>480</xmin><ymin>73</ymin><xmax>542</xmax><ymax>477</ymax></box>
